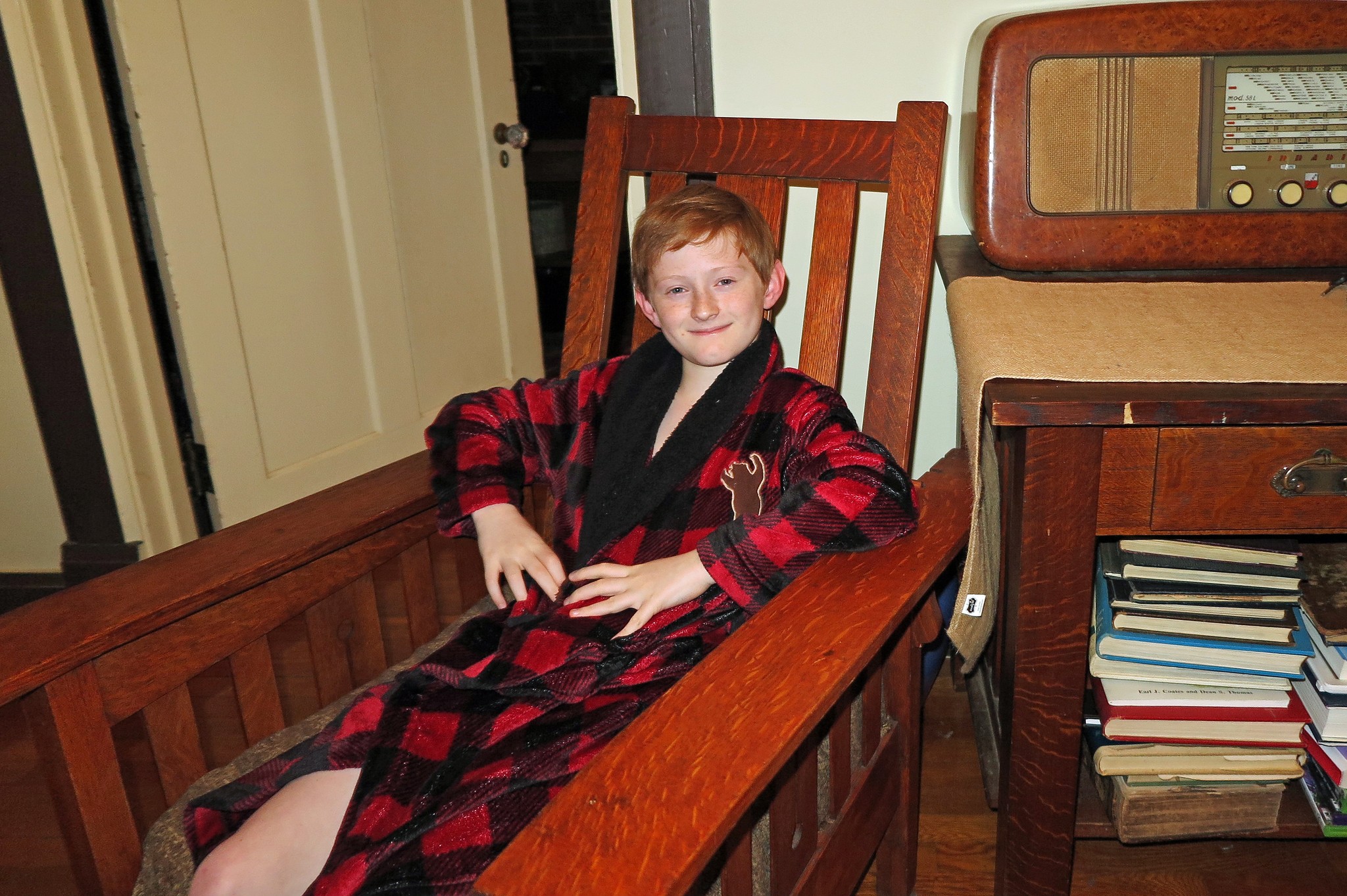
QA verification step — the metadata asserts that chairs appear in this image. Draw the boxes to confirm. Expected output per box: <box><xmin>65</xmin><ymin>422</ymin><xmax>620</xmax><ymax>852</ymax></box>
<box><xmin>0</xmin><ymin>90</ymin><xmax>946</xmax><ymax>896</ymax></box>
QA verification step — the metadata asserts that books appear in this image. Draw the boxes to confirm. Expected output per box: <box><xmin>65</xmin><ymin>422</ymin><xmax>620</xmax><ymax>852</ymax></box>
<box><xmin>1080</xmin><ymin>534</ymin><xmax>1346</xmax><ymax>842</ymax></box>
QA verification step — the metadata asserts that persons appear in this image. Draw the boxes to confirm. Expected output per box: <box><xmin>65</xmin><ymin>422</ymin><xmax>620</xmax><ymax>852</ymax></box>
<box><xmin>177</xmin><ymin>177</ymin><xmax>926</xmax><ymax>895</ymax></box>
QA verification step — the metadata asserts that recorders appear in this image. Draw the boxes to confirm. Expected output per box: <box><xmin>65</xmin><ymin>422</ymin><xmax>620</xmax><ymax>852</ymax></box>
<box><xmin>976</xmin><ymin>0</ymin><xmax>1343</xmax><ymax>282</ymax></box>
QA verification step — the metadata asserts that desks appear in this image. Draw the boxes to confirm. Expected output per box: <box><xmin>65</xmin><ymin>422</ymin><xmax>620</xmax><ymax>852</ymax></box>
<box><xmin>933</xmin><ymin>222</ymin><xmax>1343</xmax><ymax>891</ymax></box>
